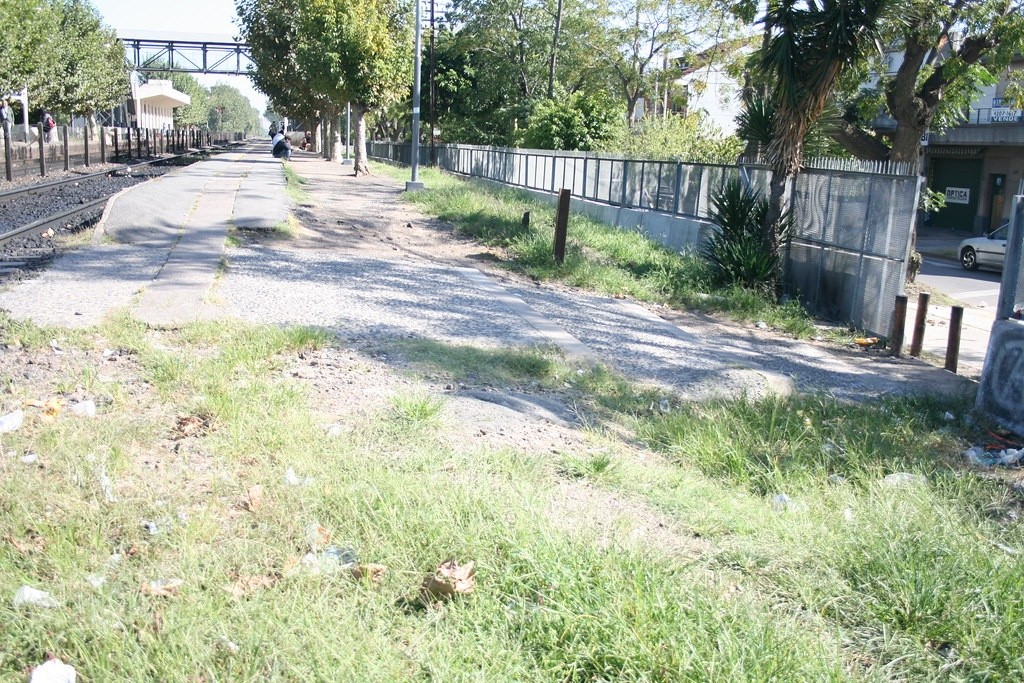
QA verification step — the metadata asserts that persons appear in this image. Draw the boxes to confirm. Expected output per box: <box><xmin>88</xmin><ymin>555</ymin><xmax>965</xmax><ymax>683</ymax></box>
<box><xmin>273</xmin><ymin>130</ymin><xmax>285</xmax><ymax>147</ymax></box>
<box><xmin>300</xmin><ymin>132</ymin><xmax>311</xmax><ymax>150</ymax></box>
<box><xmin>39</xmin><ymin>109</ymin><xmax>52</xmax><ymax>143</ymax></box>
<box><xmin>269</xmin><ymin>121</ymin><xmax>277</xmax><ymax>140</ymax></box>
<box><xmin>0</xmin><ymin>100</ymin><xmax>14</xmax><ymax>129</ymax></box>
<box><xmin>272</xmin><ymin>135</ymin><xmax>294</xmax><ymax>161</ymax></box>
<box><xmin>637</xmin><ymin>175</ymin><xmax>653</xmax><ymax>208</ymax></box>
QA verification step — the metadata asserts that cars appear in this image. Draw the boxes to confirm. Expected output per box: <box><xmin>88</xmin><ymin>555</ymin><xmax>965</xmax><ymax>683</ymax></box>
<box><xmin>959</xmin><ymin>221</ymin><xmax>1011</xmax><ymax>275</ymax></box>
